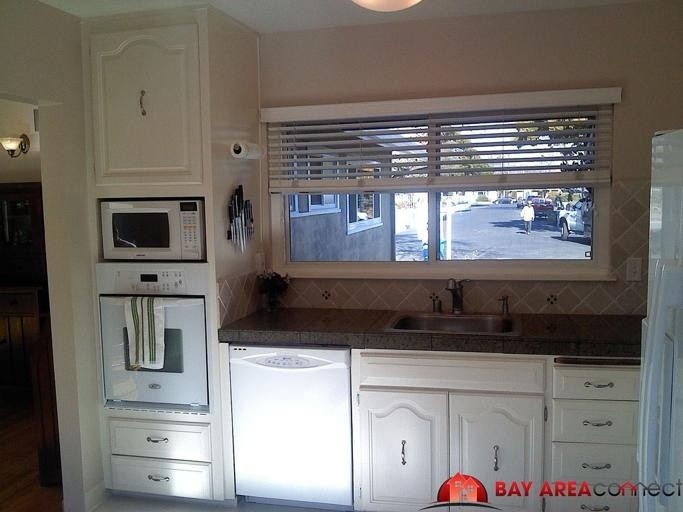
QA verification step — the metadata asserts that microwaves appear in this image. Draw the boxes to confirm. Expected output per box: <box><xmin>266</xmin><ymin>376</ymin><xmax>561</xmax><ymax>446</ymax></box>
<box><xmin>101</xmin><ymin>200</ymin><xmax>205</xmax><ymax>263</ymax></box>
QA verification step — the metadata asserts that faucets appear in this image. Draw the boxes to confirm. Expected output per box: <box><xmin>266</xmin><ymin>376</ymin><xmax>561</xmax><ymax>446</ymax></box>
<box><xmin>445</xmin><ymin>278</ymin><xmax>471</xmax><ymax>314</ymax></box>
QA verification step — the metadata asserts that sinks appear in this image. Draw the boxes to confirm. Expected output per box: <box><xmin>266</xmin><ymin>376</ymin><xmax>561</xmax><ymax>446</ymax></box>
<box><xmin>382</xmin><ymin>310</ymin><xmax>524</xmax><ymax>336</ymax></box>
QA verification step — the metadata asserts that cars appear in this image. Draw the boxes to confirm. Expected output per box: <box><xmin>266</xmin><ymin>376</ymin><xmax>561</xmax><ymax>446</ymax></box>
<box><xmin>491</xmin><ymin>193</ymin><xmax>592</xmax><ymax>241</ymax></box>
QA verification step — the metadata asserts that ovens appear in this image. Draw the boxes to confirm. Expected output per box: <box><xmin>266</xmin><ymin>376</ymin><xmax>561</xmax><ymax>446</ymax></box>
<box><xmin>101</xmin><ymin>295</ymin><xmax>208</xmax><ymax>409</ymax></box>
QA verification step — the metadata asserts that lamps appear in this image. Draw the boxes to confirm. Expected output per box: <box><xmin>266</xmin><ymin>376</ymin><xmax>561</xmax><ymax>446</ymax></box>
<box><xmin>0</xmin><ymin>131</ymin><xmax>30</xmax><ymax>159</ymax></box>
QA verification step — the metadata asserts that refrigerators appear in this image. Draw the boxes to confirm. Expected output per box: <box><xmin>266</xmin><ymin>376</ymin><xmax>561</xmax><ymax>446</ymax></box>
<box><xmin>638</xmin><ymin>123</ymin><xmax>683</xmax><ymax>512</ymax></box>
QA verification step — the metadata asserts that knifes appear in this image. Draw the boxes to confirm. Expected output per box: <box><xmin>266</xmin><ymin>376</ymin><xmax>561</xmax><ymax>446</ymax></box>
<box><xmin>227</xmin><ymin>184</ymin><xmax>256</xmax><ymax>254</ymax></box>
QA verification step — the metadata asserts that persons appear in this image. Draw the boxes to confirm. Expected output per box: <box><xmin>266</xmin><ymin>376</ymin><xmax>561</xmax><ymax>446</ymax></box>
<box><xmin>520</xmin><ymin>201</ymin><xmax>534</xmax><ymax>235</ymax></box>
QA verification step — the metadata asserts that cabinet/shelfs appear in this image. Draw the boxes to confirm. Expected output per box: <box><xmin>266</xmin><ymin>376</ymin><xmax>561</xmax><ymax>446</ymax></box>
<box><xmin>79</xmin><ymin>3</ymin><xmax>260</xmax><ymax>509</ymax></box>
